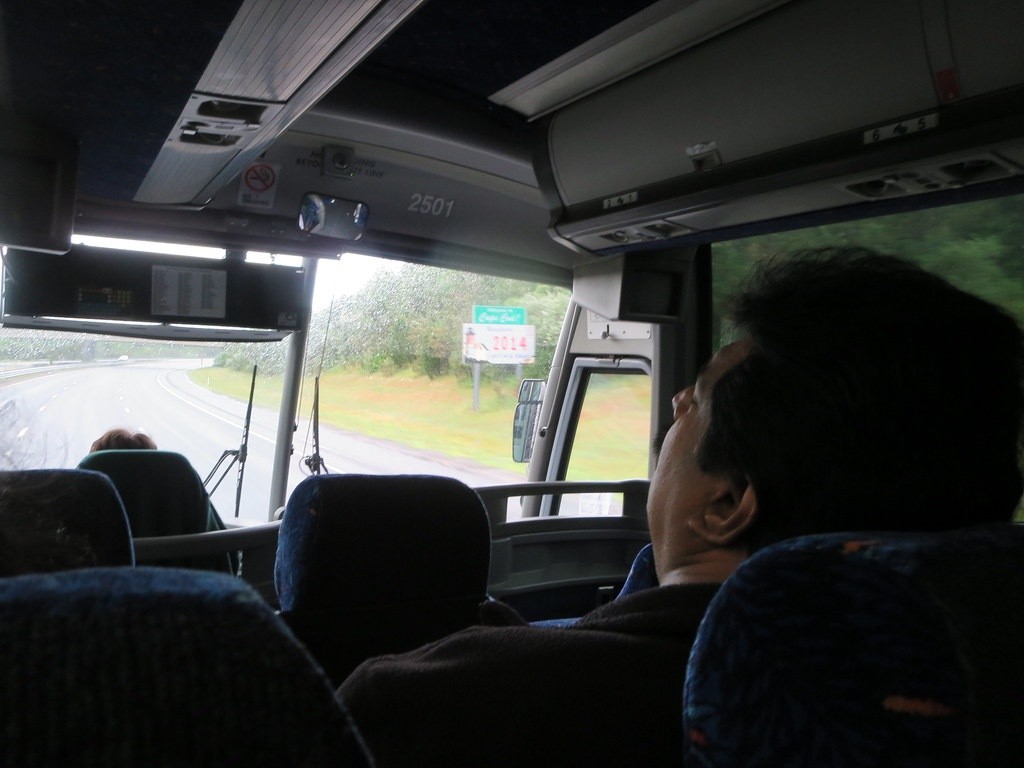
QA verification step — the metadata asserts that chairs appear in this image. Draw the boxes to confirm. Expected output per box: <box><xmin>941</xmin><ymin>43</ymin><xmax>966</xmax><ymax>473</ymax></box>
<box><xmin>0</xmin><ymin>565</ymin><xmax>381</xmax><ymax>768</ymax></box>
<box><xmin>275</xmin><ymin>473</ymin><xmax>531</xmax><ymax>693</ymax></box>
<box><xmin>0</xmin><ymin>467</ymin><xmax>139</xmax><ymax>578</ymax></box>
<box><xmin>679</xmin><ymin>527</ymin><xmax>1024</xmax><ymax>768</ymax></box>
<box><xmin>77</xmin><ymin>447</ymin><xmax>235</xmax><ymax>575</ymax></box>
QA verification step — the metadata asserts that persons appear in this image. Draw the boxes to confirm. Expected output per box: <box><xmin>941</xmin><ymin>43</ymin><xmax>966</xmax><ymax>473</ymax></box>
<box><xmin>89</xmin><ymin>429</ymin><xmax>157</xmax><ymax>453</ymax></box>
<box><xmin>335</xmin><ymin>244</ymin><xmax>1024</xmax><ymax>759</ymax></box>
